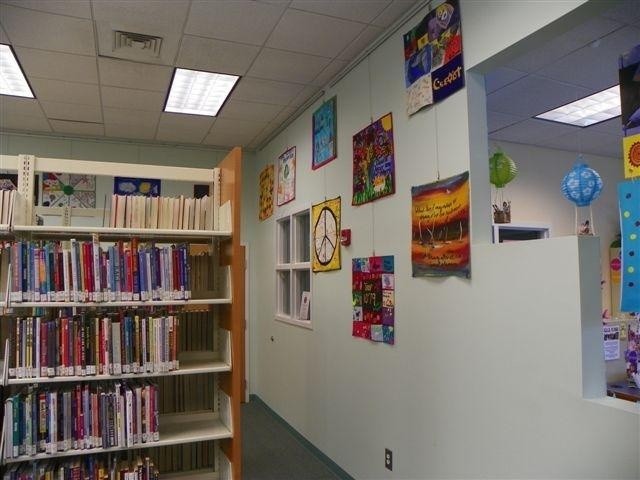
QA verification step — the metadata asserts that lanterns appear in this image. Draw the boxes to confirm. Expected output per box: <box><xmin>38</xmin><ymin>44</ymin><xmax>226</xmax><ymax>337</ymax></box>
<box><xmin>489</xmin><ymin>152</ymin><xmax>518</xmax><ymax>224</ymax></box>
<box><xmin>561</xmin><ymin>163</ymin><xmax>602</xmax><ymax>236</ymax></box>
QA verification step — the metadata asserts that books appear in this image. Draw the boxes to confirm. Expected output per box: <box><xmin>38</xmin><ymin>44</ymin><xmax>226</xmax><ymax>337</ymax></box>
<box><xmin>1</xmin><ymin>191</ymin><xmax>21</xmax><ymax>231</ymax></box>
<box><xmin>9</xmin><ymin>314</ymin><xmax>180</xmax><ymax>379</ymax></box>
<box><xmin>10</xmin><ymin>236</ymin><xmax>192</xmax><ymax>300</ymax></box>
<box><xmin>110</xmin><ymin>194</ymin><xmax>215</xmax><ymax>231</ymax></box>
<box><xmin>4</xmin><ymin>381</ymin><xmax>159</xmax><ymax>458</ymax></box>
<box><xmin>3</xmin><ymin>456</ymin><xmax>160</xmax><ymax>480</ymax></box>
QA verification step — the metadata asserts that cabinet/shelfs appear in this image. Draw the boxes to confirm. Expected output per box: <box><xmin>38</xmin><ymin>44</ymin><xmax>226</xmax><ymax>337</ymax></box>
<box><xmin>0</xmin><ymin>144</ymin><xmax>244</xmax><ymax>480</ymax></box>
